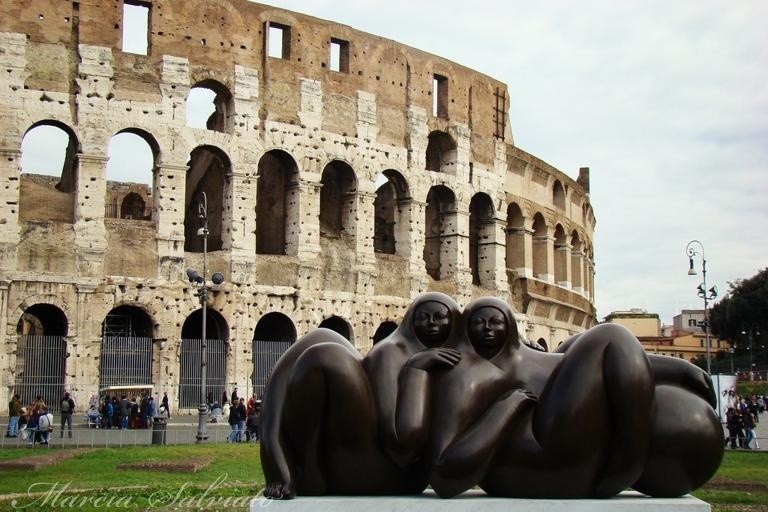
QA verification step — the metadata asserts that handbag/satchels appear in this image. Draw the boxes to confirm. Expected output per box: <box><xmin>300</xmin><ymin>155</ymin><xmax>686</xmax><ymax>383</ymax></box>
<box><xmin>48</xmin><ymin>426</ymin><xmax>53</xmax><ymax>432</ymax></box>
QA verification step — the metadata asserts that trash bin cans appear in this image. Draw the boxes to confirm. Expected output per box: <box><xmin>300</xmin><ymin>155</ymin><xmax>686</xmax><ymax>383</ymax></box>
<box><xmin>152</xmin><ymin>416</ymin><xmax>167</xmax><ymax>444</ymax></box>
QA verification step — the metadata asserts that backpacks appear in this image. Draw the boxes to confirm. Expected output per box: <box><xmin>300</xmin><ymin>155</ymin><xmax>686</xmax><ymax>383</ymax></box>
<box><xmin>62</xmin><ymin>399</ymin><xmax>70</xmax><ymax>411</ymax></box>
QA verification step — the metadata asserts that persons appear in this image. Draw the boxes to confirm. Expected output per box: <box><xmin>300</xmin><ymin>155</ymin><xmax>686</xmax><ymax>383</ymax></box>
<box><xmin>60</xmin><ymin>392</ymin><xmax>75</xmax><ymax>437</ymax></box>
<box><xmin>259</xmin><ymin>293</ymin><xmax>725</xmax><ymax>500</ymax></box>
<box><xmin>725</xmin><ymin>368</ymin><xmax>767</xmax><ymax>450</ymax></box>
<box><xmin>88</xmin><ymin>392</ymin><xmax>172</xmax><ymax>430</ymax></box>
<box><xmin>207</xmin><ymin>387</ymin><xmax>261</xmax><ymax>443</ymax></box>
<box><xmin>7</xmin><ymin>395</ymin><xmax>53</xmax><ymax>444</ymax></box>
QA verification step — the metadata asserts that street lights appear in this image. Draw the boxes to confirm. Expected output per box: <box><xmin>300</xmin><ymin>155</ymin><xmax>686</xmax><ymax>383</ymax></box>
<box><xmin>187</xmin><ymin>191</ymin><xmax>224</xmax><ymax>446</ymax></box>
<box><xmin>686</xmin><ymin>241</ymin><xmax>717</xmax><ymax>376</ymax></box>
